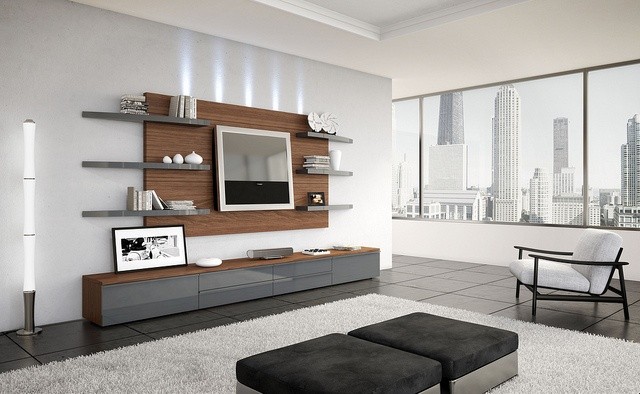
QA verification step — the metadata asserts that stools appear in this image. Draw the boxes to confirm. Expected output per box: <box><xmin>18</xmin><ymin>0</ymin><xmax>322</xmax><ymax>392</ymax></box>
<box><xmin>347</xmin><ymin>311</ymin><xmax>519</xmax><ymax>394</ymax></box>
<box><xmin>235</xmin><ymin>332</ymin><xmax>442</xmax><ymax>394</ymax></box>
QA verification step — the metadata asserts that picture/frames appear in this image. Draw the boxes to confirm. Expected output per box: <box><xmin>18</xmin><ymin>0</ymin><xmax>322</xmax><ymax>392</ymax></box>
<box><xmin>308</xmin><ymin>191</ymin><xmax>325</xmax><ymax>205</ymax></box>
<box><xmin>112</xmin><ymin>224</ymin><xmax>188</xmax><ymax>274</ymax></box>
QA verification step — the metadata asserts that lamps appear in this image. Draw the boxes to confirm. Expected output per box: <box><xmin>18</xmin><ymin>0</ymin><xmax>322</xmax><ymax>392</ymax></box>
<box><xmin>16</xmin><ymin>118</ymin><xmax>43</xmax><ymax>335</ymax></box>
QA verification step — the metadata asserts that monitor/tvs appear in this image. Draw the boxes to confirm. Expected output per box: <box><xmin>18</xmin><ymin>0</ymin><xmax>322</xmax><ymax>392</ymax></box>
<box><xmin>214</xmin><ymin>124</ymin><xmax>295</xmax><ymax>212</ymax></box>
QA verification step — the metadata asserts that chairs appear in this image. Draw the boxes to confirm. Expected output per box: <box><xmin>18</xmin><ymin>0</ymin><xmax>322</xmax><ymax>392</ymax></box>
<box><xmin>507</xmin><ymin>228</ymin><xmax>631</xmax><ymax>323</ymax></box>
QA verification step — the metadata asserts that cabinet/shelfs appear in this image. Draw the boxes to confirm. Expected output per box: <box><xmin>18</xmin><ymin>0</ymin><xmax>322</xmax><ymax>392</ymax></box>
<box><xmin>81</xmin><ymin>247</ymin><xmax>380</xmax><ymax>327</ymax></box>
<box><xmin>80</xmin><ymin>108</ymin><xmax>212</xmax><ymax>220</ymax></box>
<box><xmin>295</xmin><ymin>129</ymin><xmax>354</xmax><ymax>213</ymax></box>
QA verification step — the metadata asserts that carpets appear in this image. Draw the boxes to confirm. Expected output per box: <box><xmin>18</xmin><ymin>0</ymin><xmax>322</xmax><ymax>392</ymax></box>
<box><xmin>1</xmin><ymin>292</ymin><xmax>640</xmax><ymax>394</ymax></box>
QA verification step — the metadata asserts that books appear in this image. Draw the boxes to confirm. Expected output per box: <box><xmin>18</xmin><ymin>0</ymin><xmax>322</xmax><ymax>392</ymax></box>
<box><xmin>303</xmin><ymin>155</ymin><xmax>331</xmax><ymax>169</ymax></box>
<box><xmin>128</xmin><ymin>187</ymin><xmax>197</xmax><ymax>211</ymax></box>
<box><xmin>169</xmin><ymin>95</ymin><xmax>197</xmax><ymax>119</ymax></box>
